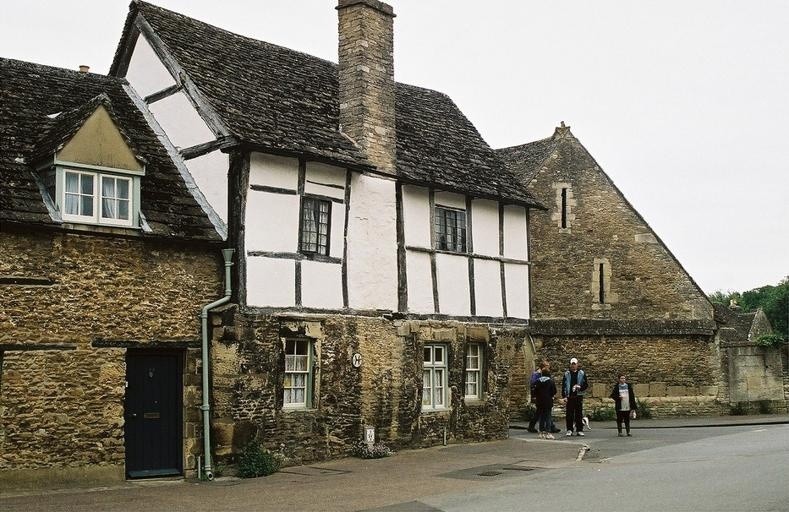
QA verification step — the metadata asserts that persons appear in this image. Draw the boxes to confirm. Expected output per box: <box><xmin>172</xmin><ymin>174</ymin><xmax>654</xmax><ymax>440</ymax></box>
<box><xmin>533</xmin><ymin>367</ymin><xmax>557</xmax><ymax>441</ymax></box>
<box><xmin>561</xmin><ymin>357</ymin><xmax>588</xmax><ymax>437</ymax></box>
<box><xmin>610</xmin><ymin>374</ymin><xmax>637</xmax><ymax>436</ymax></box>
<box><xmin>527</xmin><ymin>360</ymin><xmax>561</xmax><ymax>433</ymax></box>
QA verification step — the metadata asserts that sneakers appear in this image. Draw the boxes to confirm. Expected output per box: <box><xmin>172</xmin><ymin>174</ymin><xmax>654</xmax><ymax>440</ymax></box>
<box><xmin>528</xmin><ymin>427</ymin><xmax>561</xmax><ymax>439</ymax></box>
<box><xmin>618</xmin><ymin>432</ymin><xmax>623</xmax><ymax>436</ymax></box>
<box><xmin>577</xmin><ymin>431</ymin><xmax>584</xmax><ymax>436</ymax></box>
<box><xmin>627</xmin><ymin>433</ymin><xmax>632</xmax><ymax>436</ymax></box>
<box><xmin>566</xmin><ymin>430</ymin><xmax>572</xmax><ymax>436</ymax></box>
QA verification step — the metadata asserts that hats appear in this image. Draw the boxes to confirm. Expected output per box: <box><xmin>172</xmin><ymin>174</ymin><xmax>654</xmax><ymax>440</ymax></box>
<box><xmin>571</xmin><ymin>357</ymin><xmax>578</xmax><ymax>365</ymax></box>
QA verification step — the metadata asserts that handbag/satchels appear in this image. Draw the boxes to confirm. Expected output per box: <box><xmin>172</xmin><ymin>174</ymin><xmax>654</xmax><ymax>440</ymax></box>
<box><xmin>630</xmin><ymin>410</ymin><xmax>636</xmax><ymax>419</ymax></box>
